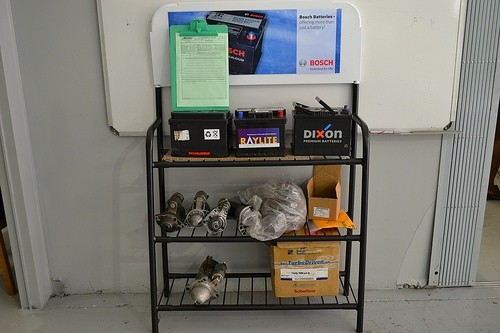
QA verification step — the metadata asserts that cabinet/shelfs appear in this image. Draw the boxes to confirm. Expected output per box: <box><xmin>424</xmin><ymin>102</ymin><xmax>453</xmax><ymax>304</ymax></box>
<box><xmin>144</xmin><ymin>0</ymin><xmax>372</xmax><ymax>332</ymax></box>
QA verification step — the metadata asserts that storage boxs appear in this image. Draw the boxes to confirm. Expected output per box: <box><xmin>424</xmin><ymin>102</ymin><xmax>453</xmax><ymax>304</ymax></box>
<box><xmin>269</xmin><ymin>239</ymin><xmax>343</xmax><ymax>298</ymax></box>
<box><xmin>304</xmin><ymin>163</ymin><xmax>341</xmax><ymax>221</ymax></box>
